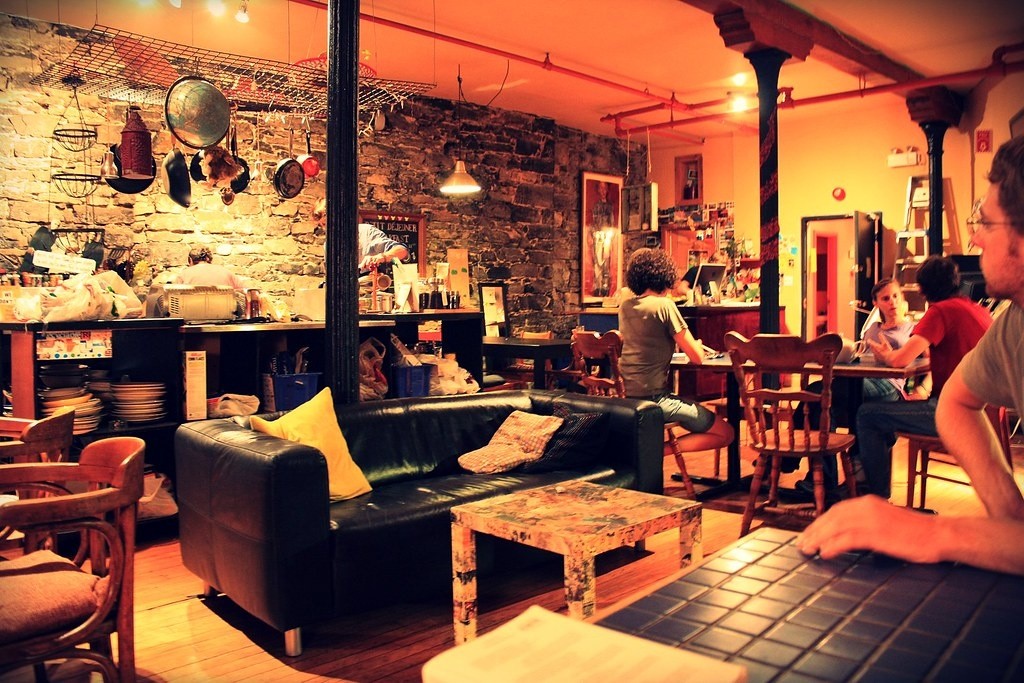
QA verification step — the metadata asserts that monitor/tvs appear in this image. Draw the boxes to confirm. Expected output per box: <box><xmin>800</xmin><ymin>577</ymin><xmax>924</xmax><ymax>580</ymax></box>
<box><xmin>692</xmin><ymin>263</ymin><xmax>726</xmax><ymax>305</ymax></box>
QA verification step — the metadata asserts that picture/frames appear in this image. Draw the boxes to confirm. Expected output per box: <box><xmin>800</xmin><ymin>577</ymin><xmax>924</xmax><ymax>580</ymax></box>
<box><xmin>578</xmin><ymin>172</ymin><xmax>625</xmax><ymax>305</ymax></box>
<box><xmin>359</xmin><ymin>207</ymin><xmax>427</xmax><ymax>276</ymax></box>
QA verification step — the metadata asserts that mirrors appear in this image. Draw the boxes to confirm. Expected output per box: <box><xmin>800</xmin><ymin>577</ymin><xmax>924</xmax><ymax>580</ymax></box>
<box><xmin>478</xmin><ymin>283</ymin><xmax>509</xmax><ymax>339</ymax></box>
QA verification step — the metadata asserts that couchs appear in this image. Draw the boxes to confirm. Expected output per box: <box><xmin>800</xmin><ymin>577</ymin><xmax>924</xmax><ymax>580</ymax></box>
<box><xmin>176</xmin><ymin>389</ymin><xmax>670</xmax><ymax>662</ymax></box>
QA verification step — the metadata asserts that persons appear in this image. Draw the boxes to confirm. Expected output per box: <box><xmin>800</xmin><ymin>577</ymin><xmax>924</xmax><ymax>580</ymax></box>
<box><xmin>181</xmin><ymin>247</ymin><xmax>243</xmax><ymax>319</ymax></box>
<box><xmin>752</xmin><ymin>279</ymin><xmax>931</xmax><ymax>494</ymax></box>
<box><xmin>619</xmin><ymin>248</ymin><xmax>734</xmax><ymax>457</ymax></box>
<box><xmin>590</xmin><ymin>182</ymin><xmax>614</xmax><ymax>297</ymax></box>
<box><xmin>311</xmin><ymin>197</ymin><xmax>408</xmax><ymax>276</ymax></box>
<box><xmin>857</xmin><ymin>255</ymin><xmax>994</xmax><ymax>498</ymax></box>
<box><xmin>678</xmin><ymin>265</ymin><xmax>699</xmax><ymax>295</ymax></box>
<box><xmin>795</xmin><ymin>133</ymin><xmax>1024</xmax><ymax>576</ymax></box>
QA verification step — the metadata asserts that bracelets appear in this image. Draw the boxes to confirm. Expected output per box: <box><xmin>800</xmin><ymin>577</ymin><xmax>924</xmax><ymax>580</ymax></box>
<box><xmin>381</xmin><ymin>252</ymin><xmax>386</xmax><ymax>262</ymax></box>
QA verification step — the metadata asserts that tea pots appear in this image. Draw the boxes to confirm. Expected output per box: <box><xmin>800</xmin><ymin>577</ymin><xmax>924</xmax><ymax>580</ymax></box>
<box><xmin>824</xmin><ymin>332</ymin><xmax>867</xmax><ymax>364</ymax></box>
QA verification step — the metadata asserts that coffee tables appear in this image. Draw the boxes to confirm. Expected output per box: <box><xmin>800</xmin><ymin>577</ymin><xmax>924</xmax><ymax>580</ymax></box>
<box><xmin>449</xmin><ymin>480</ymin><xmax>702</xmax><ymax>645</ymax></box>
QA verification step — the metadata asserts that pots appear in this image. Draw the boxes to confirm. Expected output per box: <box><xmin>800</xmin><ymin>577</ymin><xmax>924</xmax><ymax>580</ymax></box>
<box><xmin>229</xmin><ymin>123</ymin><xmax>250</xmax><ymax>194</ymax></box>
<box><xmin>190</xmin><ymin>152</ymin><xmax>217</xmax><ymax>188</ymax></box>
<box><xmin>161</xmin><ymin>133</ymin><xmax>191</xmax><ymax>209</ymax></box>
<box><xmin>273</xmin><ymin>128</ymin><xmax>305</xmax><ymax>199</ymax></box>
<box><xmin>100</xmin><ymin>107</ymin><xmax>157</xmax><ymax>194</ymax></box>
<box><xmin>296</xmin><ymin>132</ymin><xmax>320</xmax><ymax>180</ymax></box>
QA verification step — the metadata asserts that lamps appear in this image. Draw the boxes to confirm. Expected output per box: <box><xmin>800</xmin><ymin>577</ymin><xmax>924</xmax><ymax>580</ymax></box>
<box><xmin>234</xmin><ymin>0</ymin><xmax>250</xmax><ymax>24</ymax></box>
<box><xmin>440</xmin><ymin>63</ymin><xmax>482</xmax><ymax>195</ymax></box>
<box><xmin>886</xmin><ymin>144</ymin><xmax>919</xmax><ymax>169</ymax></box>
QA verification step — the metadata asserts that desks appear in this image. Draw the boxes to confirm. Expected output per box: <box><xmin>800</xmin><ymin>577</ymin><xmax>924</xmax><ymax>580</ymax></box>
<box><xmin>592</xmin><ymin>524</ymin><xmax>1024</xmax><ymax>683</ymax></box>
<box><xmin>659</xmin><ymin>356</ymin><xmax>939</xmax><ymax>517</ymax></box>
<box><xmin>480</xmin><ymin>338</ymin><xmax>571</xmax><ymax>394</ymax></box>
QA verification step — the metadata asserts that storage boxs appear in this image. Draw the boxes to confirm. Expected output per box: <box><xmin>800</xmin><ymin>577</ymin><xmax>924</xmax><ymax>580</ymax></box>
<box><xmin>385</xmin><ymin>363</ymin><xmax>435</xmax><ymax>397</ymax></box>
<box><xmin>258</xmin><ymin>372</ymin><xmax>322</xmax><ymax>408</ymax></box>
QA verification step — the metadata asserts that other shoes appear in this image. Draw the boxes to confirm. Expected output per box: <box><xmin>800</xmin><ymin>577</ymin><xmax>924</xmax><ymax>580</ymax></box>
<box><xmin>777</xmin><ymin>488</ymin><xmax>815</xmax><ymax>503</ymax></box>
<box><xmin>752</xmin><ymin>456</ymin><xmax>794</xmax><ymax>474</ymax></box>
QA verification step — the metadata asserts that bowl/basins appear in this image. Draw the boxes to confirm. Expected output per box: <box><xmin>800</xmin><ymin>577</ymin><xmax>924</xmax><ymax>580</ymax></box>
<box><xmin>164</xmin><ymin>76</ymin><xmax>231</xmax><ymax>150</ymax></box>
<box><xmin>39</xmin><ymin>364</ymin><xmax>109</xmax><ymax>389</ymax></box>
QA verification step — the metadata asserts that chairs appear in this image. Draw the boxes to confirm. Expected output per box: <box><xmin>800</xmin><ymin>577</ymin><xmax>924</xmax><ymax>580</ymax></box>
<box><xmin>0</xmin><ymin>404</ymin><xmax>147</xmax><ymax>683</ymax></box>
<box><xmin>513</xmin><ymin>317</ymin><xmax>1024</xmax><ymax>541</ymax></box>
<box><xmin>507</xmin><ymin>330</ymin><xmax>551</xmax><ymax>389</ymax></box>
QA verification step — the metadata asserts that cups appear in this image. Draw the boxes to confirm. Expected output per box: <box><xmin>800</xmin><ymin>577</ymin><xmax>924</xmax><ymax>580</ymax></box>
<box><xmin>376</xmin><ymin>273</ymin><xmax>392</xmax><ymax>291</ymax></box>
<box><xmin>445</xmin><ymin>290</ymin><xmax>459</xmax><ymax>309</ymax></box>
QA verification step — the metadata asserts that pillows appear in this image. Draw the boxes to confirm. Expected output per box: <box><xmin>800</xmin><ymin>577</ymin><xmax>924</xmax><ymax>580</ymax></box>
<box><xmin>249</xmin><ymin>387</ymin><xmax>373</xmax><ymax>502</ymax></box>
<box><xmin>457</xmin><ymin>403</ymin><xmax>610</xmax><ymax>476</ymax></box>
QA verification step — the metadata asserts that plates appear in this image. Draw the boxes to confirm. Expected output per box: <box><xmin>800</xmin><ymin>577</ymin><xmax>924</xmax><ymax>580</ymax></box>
<box><xmin>36</xmin><ymin>381</ymin><xmax>168</xmax><ymax>436</ymax></box>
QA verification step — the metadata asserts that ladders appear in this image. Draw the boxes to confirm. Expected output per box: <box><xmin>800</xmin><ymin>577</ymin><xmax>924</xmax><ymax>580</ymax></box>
<box><xmin>891</xmin><ymin>175</ymin><xmax>929</xmax><ymax>321</ymax></box>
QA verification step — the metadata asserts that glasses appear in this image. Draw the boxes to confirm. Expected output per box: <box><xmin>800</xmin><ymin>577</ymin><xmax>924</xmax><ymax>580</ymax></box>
<box><xmin>967</xmin><ymin>218</ymin><xmax>1015</xmax><ymax>236</ymax></box>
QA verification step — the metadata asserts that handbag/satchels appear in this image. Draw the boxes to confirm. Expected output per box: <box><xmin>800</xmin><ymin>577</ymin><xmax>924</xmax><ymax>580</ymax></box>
<box><xmin>44</xmin><ymin>271</ymin><xmax>114</xmax><ymax>323</ymax></box>
<box><xmin>136</xmin><ymin>471</ymin><xmax>180</xmax><ymax>521</ymax></box>
